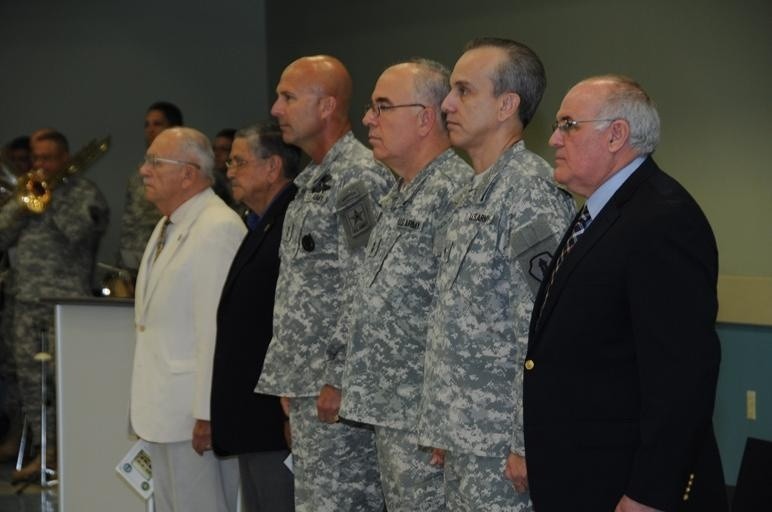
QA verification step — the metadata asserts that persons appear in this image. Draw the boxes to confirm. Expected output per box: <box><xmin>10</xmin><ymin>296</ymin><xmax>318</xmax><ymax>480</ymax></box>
<box><xmin>1</xmin><ymin>129</ymin><xmax>108</xmax><ymax>484</ymax></box>
<box><xmin>252</xmin><ymin>54</ymin><xmax>396</xmax><ymax>512</ymax></box>
<box><xmin>412</xmin><ymin>38</ymin><xmax>577</xmax><ymax>512</ymax></box>
<box><xmin>1</xmin><ymin>135</ymin><xmax>31</xmax><ymax>462</ymax></box>
<box><xmin>210</xmin><ymin>128</ymin><xmax>245</xmax><ymax>221</ymax></box>
<box><xmin>334</xmin><ymin>61</ymin><xmax>477</xmax><ymax>512</ymax></box>
<box><xmin>129</xmin><ymin>128</ymin><xmax>249</xmax><ymax>512</ymax></box>
<box><xmin>120</xmin><ymin>103</ymin><xmax>183</xmax><ymax>280</ymax></box>
<box><xmin>209</xmin><ymin>114</ymin><xmax>303</xmax><ymax>511</ymax></box>
<box><xmin>523</xmin><ymin>73</ymin><xmax>727</xmax><ymax>512</ymax></box>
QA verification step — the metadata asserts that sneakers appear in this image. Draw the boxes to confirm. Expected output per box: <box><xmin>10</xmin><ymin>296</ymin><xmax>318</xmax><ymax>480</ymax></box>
<box><xmin>13</xmin><ymin>444</ymin><xmax>58</xmax><ymax>480</ymax></box>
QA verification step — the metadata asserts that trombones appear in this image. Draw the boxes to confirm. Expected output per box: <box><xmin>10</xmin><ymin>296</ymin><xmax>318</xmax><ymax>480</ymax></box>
<box><xmin>17</xmin><ymin>138</ymin><xmax>110</xmax><ymax>214</ymax></box>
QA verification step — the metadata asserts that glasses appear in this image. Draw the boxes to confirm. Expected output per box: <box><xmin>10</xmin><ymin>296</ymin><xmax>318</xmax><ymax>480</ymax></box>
<box><xmin>550</xmin><ymin>117</ymin><xmax>616</xmax><ymax>134</ymax></box>
<box><xmin>142</xmin><ymin>154</ymin><xmax>201</xmax><ymax>173</ymax></box>
<box><xmin>223</xmin><ymin>156</ymin><xmax>271</xmax><ymax>170</ymax></box>
<box><xmin>361</xmin><ymin>100</ymin><xmax>425</xmax><ymax>118</ymax></box>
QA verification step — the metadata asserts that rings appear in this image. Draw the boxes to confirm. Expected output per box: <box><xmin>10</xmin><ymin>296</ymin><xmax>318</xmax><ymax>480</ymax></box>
<box><xmin>206</xmin><ymin>444</ymin><xmax>211</xmax><ymax>447</ymax></box>
<box><xmin>332</xmin><ymin>415</ymin><xmax>338</xmax><ymax>421</ymax></box>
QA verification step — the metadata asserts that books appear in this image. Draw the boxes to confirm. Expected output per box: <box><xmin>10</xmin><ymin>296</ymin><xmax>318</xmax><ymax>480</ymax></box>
<box><xmin>115</xmin><ymin>438</ymin><xmax>154</xmax><ymax>499</ymax></box>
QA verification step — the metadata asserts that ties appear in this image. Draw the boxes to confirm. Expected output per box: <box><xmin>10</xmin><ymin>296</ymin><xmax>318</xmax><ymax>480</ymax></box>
<box><xmin>150</xmin><ymin>216</ymin><xmax>172</xmax><ymax>264</ymax></box>
<box><xmin>527</xmin><ymin>203</ymin><xmax>592</xmax><ymax>346</ymax></box>
